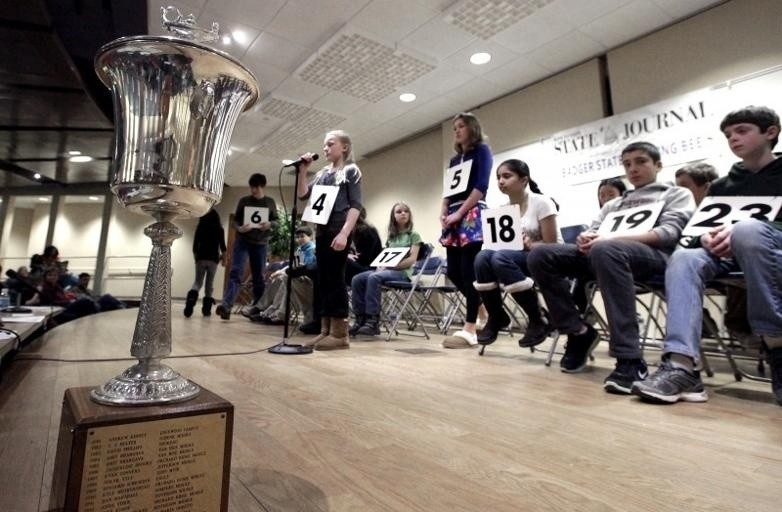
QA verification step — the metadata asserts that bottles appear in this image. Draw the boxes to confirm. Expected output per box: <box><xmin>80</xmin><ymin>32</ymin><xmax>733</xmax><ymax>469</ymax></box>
<box><xmin>0</xmin><ymin>288</ymin><xmax>10</xmax><ymax>310</ymax></box>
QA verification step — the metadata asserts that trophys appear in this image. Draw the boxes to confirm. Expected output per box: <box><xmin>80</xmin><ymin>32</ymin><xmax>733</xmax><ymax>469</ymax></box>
<box><xmin>90</xmin><ymin>3</ymin><xmax>262</xmax><ymax>409</ymax></box>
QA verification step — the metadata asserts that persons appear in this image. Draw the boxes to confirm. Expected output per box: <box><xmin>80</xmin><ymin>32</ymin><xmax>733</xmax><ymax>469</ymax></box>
<box><xmin>671</xmin><ymin>159</ymin><xmax>763</xmax><ymax>351</ymax></box>
<box><xmin>214</xmin><ymin>172</ymin><xmax>281</xmax><ymax>321</ymax></box>
<box><xmin>627</xmin><ymin>103</ymin><xmax>782</xmax><ymax>408</ymax></box>
<box><xmin>0</xmin><ymin>245</ymin><xmax>128</xmax><ymax>320</ymax></box>
<box><xmin>437</xmin><ymin>111</ymin><xmax>495</xmax><ymax>350</ymax></box>
<box><xmin>183</xmin><ymin>207</ymin><xmax>229</xmax><ymax>318</ymax></box>
<box><xmin>472</xmin><ymin>157</ymin><xmax>568</xmax><ymax>348</ymax></box>
<box><xmin>284</xmin><ymin>204</ymin><xmax>382</xmax><ymax>336</ymax></box>
<box><xmin>294</xmin><ymin>129</ymin><xmax>364</xmax><ymax>352</ymax></box>
<box><xmin>348</xmin><ymin>199</ymin><xmax>424</xmax><ymax>337</ymax></box>
<box><xmin>239</xmin><ymin>225</ymin><xmax>316</xmax><ymax>317</ymax></box>
<box><xmin>523</xmin><ymin>139</ymin><xmax>700</xmax><ymax>397</ymax></box>
<box><xmin>571</xmin><ymin>176</ymin><xmax>628</xmax><ymax>326</ymax></box>
<box><xmin>249</xmin><ymin>247</ymin><xmax>317</xmax><ymax>325</ymax></box>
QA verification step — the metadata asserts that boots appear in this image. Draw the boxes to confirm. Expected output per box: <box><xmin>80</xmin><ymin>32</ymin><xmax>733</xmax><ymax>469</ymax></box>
<box><xmin>502</xmin><ymin>277</ymin><xmax>556</xmax><ymax>347</ymax></box>
<box><xmin>203</xmin><ymin>297</ymin><xmax>215</xmax><ymax>315</ymax></box>
<box><xmin>184</xmin><ymin>290</ymin><xmax>198</xmax><ymax>317</ymax></box>
<box><xmin>299</xmin><ymin>312</ymin><xmax>381</xmax><ymax>351</ymax></box>
<box><xmin>473</xmin><ymin>280</ymin><xmax>511</xmax><ymax>345</ymax></box>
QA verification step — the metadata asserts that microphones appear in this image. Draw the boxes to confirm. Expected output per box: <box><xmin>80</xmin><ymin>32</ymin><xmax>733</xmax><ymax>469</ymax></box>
<box><xmin>293</xmin><ymin>153</ymin><xmax>319</xmax><ymax>166</ymax></box>
<box><xmin>6</xmin><ymin>269</ymin><xmax>38</xmax><ymax>292</ymax></box>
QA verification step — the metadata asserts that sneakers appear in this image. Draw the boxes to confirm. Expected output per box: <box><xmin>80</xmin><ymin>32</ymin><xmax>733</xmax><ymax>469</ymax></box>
<box><xmin>242</xmin><ymin>304</ymin><xmax>286</xmax><ymax>325</ymax></box>
<box><xmin>604</xmin><ymin>353</ymin><xmax>708</xmax><ymax>402</ymax></box>
<box><xmin>443</xmin><ymin>331</ymin><xmax>478</xmax><ymax>348</ymax></box>
<box><xmin>216</xmin><ymin>305</ymin><xmax>230</xmax><ymax>320</ymax></box>
<box><xmin>560</xmin><ymin>321</ymin><xmax>601</xmax><ymax>373</ymax></box>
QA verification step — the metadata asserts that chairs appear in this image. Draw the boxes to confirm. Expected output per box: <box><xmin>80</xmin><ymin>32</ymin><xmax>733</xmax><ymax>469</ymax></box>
<box><xmin>479</xmin><ymin>272</ymin><xmax>782</xmax><ymax>383</ymax></box>
<box><xmin>230</xmin><ymin>242</ymin><xmax>466</xmax><ymax>342</ymax></box>
